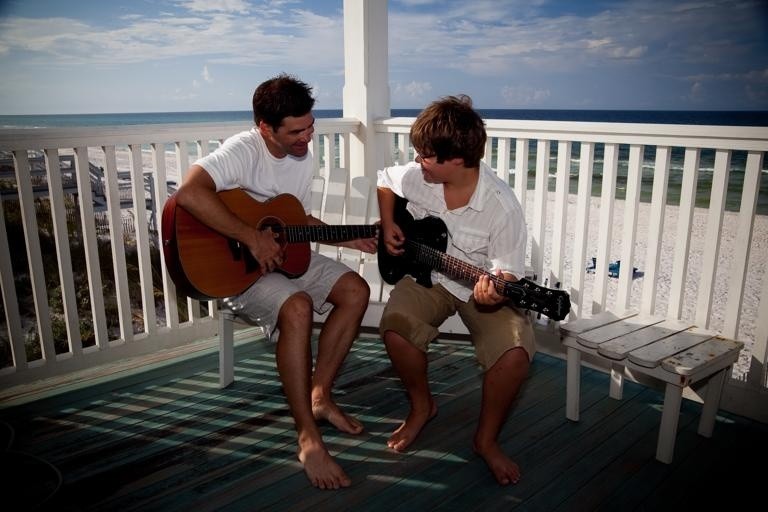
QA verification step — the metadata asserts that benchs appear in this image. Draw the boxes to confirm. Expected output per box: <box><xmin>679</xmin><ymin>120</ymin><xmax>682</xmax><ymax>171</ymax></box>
<box><xmin>215</xmin><ymin>167</ymin><xmax>536</xmax><ymax>390</ymax></box>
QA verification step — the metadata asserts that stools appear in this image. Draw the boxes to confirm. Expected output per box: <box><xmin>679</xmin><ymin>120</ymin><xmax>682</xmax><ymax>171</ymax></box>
<box><xmin>558</xmin><ymin>310</ymin><xmax>744</xmax><ymax>465</ymax></box>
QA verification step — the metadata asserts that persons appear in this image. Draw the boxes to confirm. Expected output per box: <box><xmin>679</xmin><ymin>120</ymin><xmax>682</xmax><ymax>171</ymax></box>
<box><xmin>172</xmin><ymin>70</ymin><xmax>381</xmax><ymax>493</ymax></box>
<box><xmin>366</xmin><ymin>89</ymin><xmax>537</xmax><ymax>484</ymax></box>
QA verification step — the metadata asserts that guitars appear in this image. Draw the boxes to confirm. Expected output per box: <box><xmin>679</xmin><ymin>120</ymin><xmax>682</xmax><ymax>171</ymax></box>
<box><xmin>378</xmin><ymin>203</ymin><xmax>570</xmax><ymax>321</ymax></box>
<box><xmin>161</xmin><ymin>181</ymin><xmax>379</xmax><ymax>300</ymax></box>
